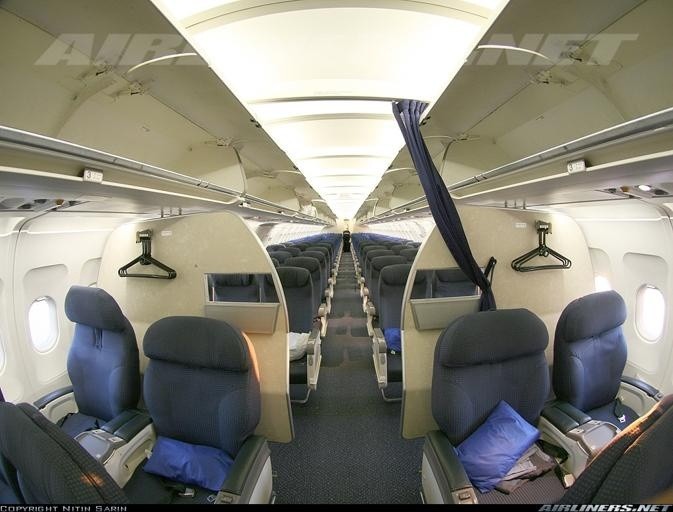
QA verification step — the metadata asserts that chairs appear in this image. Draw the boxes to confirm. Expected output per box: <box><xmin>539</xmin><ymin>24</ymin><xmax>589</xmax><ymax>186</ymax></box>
<box><xmin>420</xmin><ymin>290</ymin><xmax>672</xmax><ymax>504</ymax></box>
<box><xmin>0</xmin><ymin>286</ymin><xmax>276</xmax><ymax>504</ymax></box>
<box><xmin>207</xmin><ymin>232</ymin><xmax>477</xmax><ymax>404</ymax></box>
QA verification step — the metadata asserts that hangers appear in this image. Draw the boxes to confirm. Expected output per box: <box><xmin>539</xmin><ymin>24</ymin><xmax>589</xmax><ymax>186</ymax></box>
<box><xmin>118</xmin><ymin>237</ymin><xmax>177</xmax><ymax>279</ymax></box>
<box><xmin>511</xmin><ymin>228</ymin><xmax>571</xmax><ymax>272</ymax></box>
